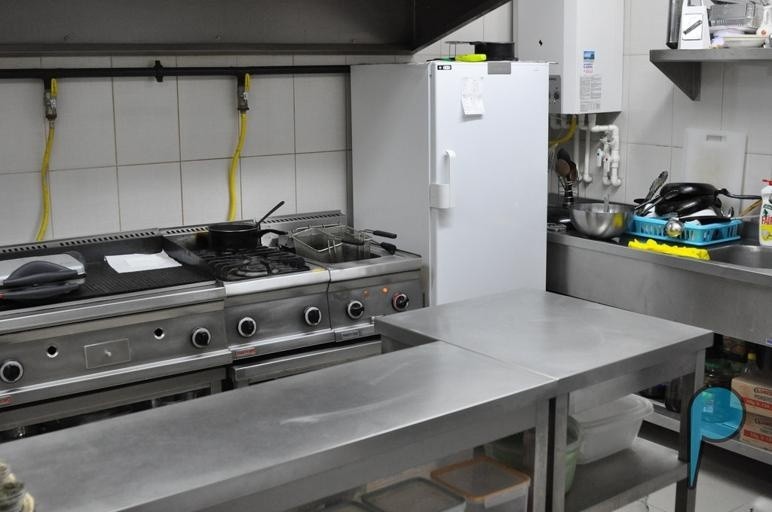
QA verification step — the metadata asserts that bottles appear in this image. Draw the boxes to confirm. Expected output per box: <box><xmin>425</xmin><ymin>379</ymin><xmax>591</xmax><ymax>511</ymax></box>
<box><xmin>757</xmin><ymin>7</ymin><xmax>771</xmax><ymax>46</ymax></box>
<box><xmin>0</xmin><ymin>463</ymin><xmax>34</xmax><ymax>510</ymax></box>
<box><xmin>638</xmin><ymin>336</ymin><xmax>760</xmax><ymax>419</ymax></box>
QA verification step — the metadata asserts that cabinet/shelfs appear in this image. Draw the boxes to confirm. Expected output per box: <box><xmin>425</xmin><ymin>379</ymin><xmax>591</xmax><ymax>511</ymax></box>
<box><xmin>0</xmin><ymin>333</ymin><xmax>559</xmax><ymax>511</ymax></box>
<box><xmin>545</xmin><ymin>221</ymin><xmax>772</xmax><ymax>469</ymax></box>
<box><xmin>363</xmin><ymin>282</ymin><xmax>719</xmax><ymax>512</ymax></box>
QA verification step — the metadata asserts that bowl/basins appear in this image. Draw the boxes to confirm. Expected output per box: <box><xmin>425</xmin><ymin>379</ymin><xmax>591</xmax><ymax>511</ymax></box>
<box><xmin>571</xmin><ymin>203</ymin><xmax>635</xmax><ymax>238</ymax></box>
<box><xmin>486</xmin><ymin>413</ymin><xmax>584</xmax><ymax>499</ymax></box>
<box><xmin>473</xmin><ymin>42</ymin><xmax>516</xmax><ymax>62</ymax></box>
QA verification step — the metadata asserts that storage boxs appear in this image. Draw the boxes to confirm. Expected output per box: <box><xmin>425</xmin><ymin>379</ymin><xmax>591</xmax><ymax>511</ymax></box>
<box><xmin>431</xmin><ymin>452</ymin><xmax>533</xmax><ymax>512</ymax></box>
<box><xmin>483</xmin><ymin>433</ymin><xmax>581</xmax><ymax>496</ymax></box>
<box><xmin>573</xmin><ymin>391</ymin><xmax>656</xmax><ymax>465</ymax></box>
<box><xmin>362</xmin><ymin>473</ymin><xmax>467</xmax><ymax>511</ymax></box>
<box><xmin>740</xmin><ymin>412</ymin><xmax>771</xmax><ymax>453</ymax></box>
<box><xmin>728</xmin><ymin>374</ymin><xmax>772</xmax><ymax>416</ymax></box>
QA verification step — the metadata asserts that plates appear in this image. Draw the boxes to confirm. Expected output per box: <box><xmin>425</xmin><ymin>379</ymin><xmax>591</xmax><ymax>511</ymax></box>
<box><xmin>721</xmin><ymin>35</ymin><xmax>766</xmax><ymax>47</ymax></box>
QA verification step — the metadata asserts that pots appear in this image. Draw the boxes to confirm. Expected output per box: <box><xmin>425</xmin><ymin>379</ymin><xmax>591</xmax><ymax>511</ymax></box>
<box><xmin>205</xmin><ymin>222</ymin><xmax>289</xmax><ymax>250</ymax></box>
<box><xmin>635</xmin><ymin>175</ymin><xmax>760</xmax><ymax>223</ymax></box>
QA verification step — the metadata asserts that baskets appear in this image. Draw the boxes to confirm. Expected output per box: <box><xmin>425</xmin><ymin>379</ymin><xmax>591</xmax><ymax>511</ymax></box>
<box><xmin>630</xmin><ymin>212</ymin><xmax>744</xmax><ymax>247</ymax></box>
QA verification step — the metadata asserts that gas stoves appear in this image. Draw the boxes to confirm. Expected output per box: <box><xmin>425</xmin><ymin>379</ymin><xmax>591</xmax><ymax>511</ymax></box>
<box><xmin>188</xmin><ymin>241</ymin><xmax>333</xmax><ymax>358</ymax></box>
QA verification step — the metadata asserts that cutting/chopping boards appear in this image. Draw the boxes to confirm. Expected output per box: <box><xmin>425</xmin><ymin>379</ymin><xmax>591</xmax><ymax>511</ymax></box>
<box><xmin>682</xmin><ymin>128</ymin><xmax>747</xmax><ymax>215</ymax></box>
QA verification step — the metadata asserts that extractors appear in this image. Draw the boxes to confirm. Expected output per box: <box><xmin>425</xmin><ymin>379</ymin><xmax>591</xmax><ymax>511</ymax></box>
<box><xmin>2</xmin><ymin>0</ymin><xmax>516</xmax><ymax>69</ymax></box>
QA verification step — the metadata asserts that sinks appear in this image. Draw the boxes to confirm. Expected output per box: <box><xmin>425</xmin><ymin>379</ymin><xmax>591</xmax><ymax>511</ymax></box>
<box><xmin>706</xmin><ymin>244</ymin><xmax>772</xmax><ymax>275</ymax></box>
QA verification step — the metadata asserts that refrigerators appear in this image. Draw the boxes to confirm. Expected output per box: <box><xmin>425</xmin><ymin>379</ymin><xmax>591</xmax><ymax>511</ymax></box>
<box><xmin>348</xmin><ymin>61</ymin><xmax>550</xmax><ymax>310</ymax></box>
